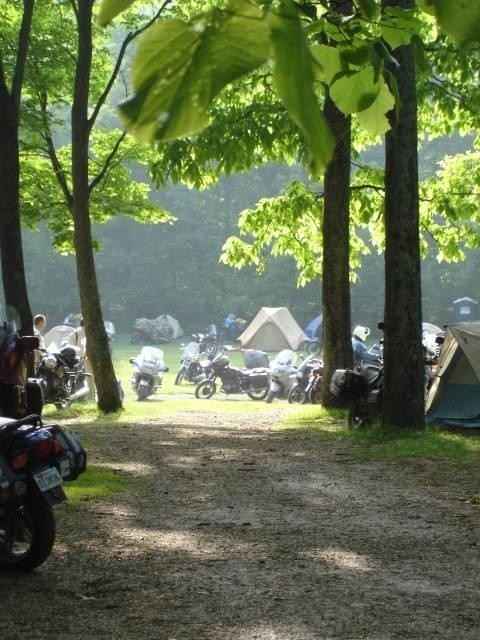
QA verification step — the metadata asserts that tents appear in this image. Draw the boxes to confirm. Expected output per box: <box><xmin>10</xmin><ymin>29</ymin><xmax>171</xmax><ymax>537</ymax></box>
<box><xmin>43</xmin><ymin>325</ymin><xmax>76</xmax><ymax>352</ymax></box>
<box><xmin>236</xmin><ymin>306</ymin><xmax>308</xmax><ymax>352</ymax></box>
<box><xmin>419</xmin><ymin>322</ymin><xmax>444</xmax><ymax>371</ymax></box>
<box><xmin>422</xmin><ymin>319</ymin><xmax>479</xmax><ymax>431</ymax></box>
<box><xmin>303</xmin><ymin>311</ymin><xmax>326</xmax><ymax>343</ymax></box>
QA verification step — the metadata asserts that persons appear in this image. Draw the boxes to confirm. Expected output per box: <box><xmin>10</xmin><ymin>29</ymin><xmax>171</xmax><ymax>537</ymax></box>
<box><xmin>63</xmin><ymin>318</ymin><xmax>116</xmax><ymax>408</ymax></box>
<box><xmin>351</xmin><ymin>325</ymin><xmax>380</xmax><ymax>369</ymax></box>
<box><xmin>32</xmin><ymin>314</ymin><xmax>47</xmax><ymax>372</ymax></box>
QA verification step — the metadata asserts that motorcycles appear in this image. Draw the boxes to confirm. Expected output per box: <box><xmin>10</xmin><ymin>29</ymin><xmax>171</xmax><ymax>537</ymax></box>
<box><xmin>1</xmin><ymin>333</ymin><xmax>101</xmax><ymax>576</ymax></box>
<box><xmin>33</xmin><ymin>343</ymin><xmax>89</xmax><ymax>414</ymax></box>
